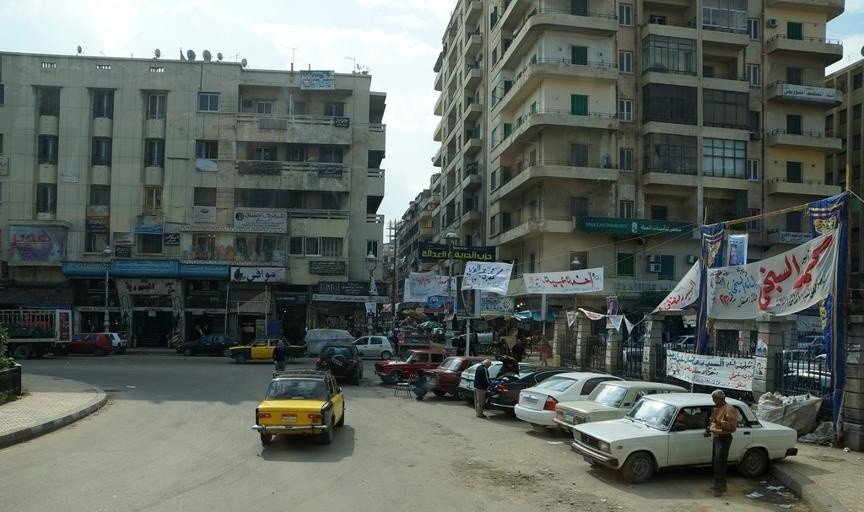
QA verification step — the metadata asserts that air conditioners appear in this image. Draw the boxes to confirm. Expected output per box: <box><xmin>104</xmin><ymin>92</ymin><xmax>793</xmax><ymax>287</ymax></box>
<box><xmin>648</xmin><ymin>264</ymin><xmax>661</xmax><ymax>272</ymax></box>
<box><xmin>649</xmin><ymin>255</ymin><xmax>661</xmax><ymax>262</ymax></box>
<box><xmin>687</xmin><ymin>255</ymin><xmax>698</xmax><ymax>264</ymax></box>
<box><xmin>768</xmin><ymin>19</ymin><xmax>779</xmax><ymax>26</ymax></box>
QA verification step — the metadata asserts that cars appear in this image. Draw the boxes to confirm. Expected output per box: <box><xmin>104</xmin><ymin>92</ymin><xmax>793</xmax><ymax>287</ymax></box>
<box><xmin>569</xmin><ymin>392</ymin><xmax>800</xmax><ymax>485</ymax></box>
<box><xmin>174</xmin><ymin>323</ymin><xmax>307</xmax><ymax>361</ymax></box>
<box><xmin>351</xmin><ymin>335</ymin><xmax>393</xmax><ymax>361</ymax></box>
<box><xmin>423</xmin><ymin>356</ymin><xmax>496</xmax><ymax>402</ymax></box>
<box><xmin>486</xmin><ymin>366</ymin><xmax>582</xmax><ymax>418</ymax></box>
<box><xmin>252</xmin><ymin>368</ymin><xmax>347</xmax><ymax>449</ymax></box>
<box><xmin>375</xmin><ymin>348</ymin><xmax>454</xmax><ymax>387</ymax></box>
<box><xmin>514</xmin><ymin>371</ymin><xmax>626</xmax><ymax>433</ymax></box>
<box><xmin>55</xmin><ymin>330</ymin><xmax>126</xmax><ymax>355</ymax></box>
<box><xmin>551</xmin><ymin>379</ymin><xmax>692</xmax><ymax>434</ymax></box>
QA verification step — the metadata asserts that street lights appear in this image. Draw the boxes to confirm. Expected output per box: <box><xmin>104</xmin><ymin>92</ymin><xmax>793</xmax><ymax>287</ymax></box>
<box><xmin>365</xmin><ymin>250</ymin><xmax>377</xmax><ymax>293</ymax></box>
<box><xmin>100</xmin><ymin>245</ymin><xmax>114</xmax><ymax>333</ymax></box>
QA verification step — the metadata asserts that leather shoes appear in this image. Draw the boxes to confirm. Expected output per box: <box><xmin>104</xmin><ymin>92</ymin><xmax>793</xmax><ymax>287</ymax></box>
<box><xmin>709</xmin><ymin>487</ymin><xmax>728</xmax><ymax>497</ymax></box>
<box><xmin>476</xmin><ymin>414</ymin><xmax>487</xmax><ymax>418</ymax></box>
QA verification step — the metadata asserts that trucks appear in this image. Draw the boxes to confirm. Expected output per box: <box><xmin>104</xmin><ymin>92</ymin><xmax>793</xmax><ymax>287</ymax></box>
<box><xmin>0</xmin><ymin>306</ymin><xmax>74</xmax><ymax>359</ymax></box>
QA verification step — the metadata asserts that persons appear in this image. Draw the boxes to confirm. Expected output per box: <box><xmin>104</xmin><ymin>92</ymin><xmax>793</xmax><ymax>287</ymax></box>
<box><xmin>409</xmin><ymin>370</ymin><xmax>428</xmax><ymax>400</ymax></box>
<box><xmin>459</xmin><ymin>333</ymin><xmax>480</xmax><ymax>356</ymax></box>
<box><xmin>540</xmin><ymin>335</ymin><xmax>550</xmax><ymax>366</ymax></box>
<box><xmin>512</xmin><ymin>338</ymin><xmax>525</xmax><ymax>363</ymax></box>
<box><xmin>707</xmin><ymin>388</ymin><xmax>737</xmax><ymax>497</ymax></box>
<box><xmin>474</xmin><ymin>359</ymin><xmax>491</xmax><ymax>417</ymax></box>
<box><xmin>272</xmin><ymin>340</ymin><xmax>289</xmax><ymax>371</ymax></box>
<box><xmin>496</xmin><ymin>338</ymin><xmax>510</xmax><ymax>359</ymax></box>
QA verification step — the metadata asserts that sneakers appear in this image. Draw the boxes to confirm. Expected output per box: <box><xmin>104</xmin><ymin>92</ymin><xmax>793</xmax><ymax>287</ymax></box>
<box><xmin>415</xmin><ymin>396</ymin><xmax>424</xmax><ymax>401</ymax></box>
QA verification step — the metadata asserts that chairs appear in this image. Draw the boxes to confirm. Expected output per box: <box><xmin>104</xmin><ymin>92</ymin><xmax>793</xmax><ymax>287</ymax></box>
<box><xmin>393</xmin><ymin>372</ymin><xmax>413</xmax><ymax>398</ymax></box>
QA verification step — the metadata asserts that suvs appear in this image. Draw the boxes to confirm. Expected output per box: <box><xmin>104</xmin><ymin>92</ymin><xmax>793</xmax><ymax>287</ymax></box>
<box><xmin>315</xmin><ymin>343</ymin><xmax>367</xmax><ymax>386</ymax></box>
<box><xmin>456</xmin><ymin>360</ymin><xmax>539</xmax><ymax>405</ymax></box>
